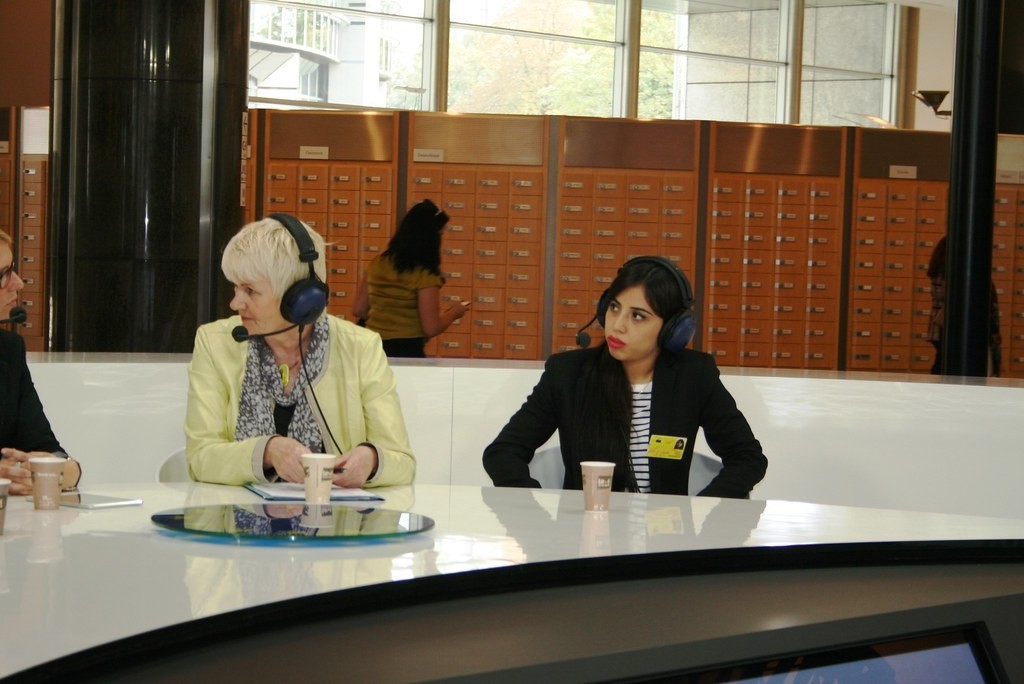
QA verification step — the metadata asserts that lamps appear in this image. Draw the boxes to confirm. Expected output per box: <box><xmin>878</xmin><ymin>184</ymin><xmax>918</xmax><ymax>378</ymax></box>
<box><xmin>911</xmin><ymin>91</ymin><xmax>951</xmax><ymax>120</ymax></box>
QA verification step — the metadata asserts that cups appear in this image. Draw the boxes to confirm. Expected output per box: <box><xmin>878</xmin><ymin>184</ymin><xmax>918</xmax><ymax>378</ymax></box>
<box><xmin>300</xmin><ymin>453</ymin><xmax>337</xmax><ymax>504</ymax></box>
<box><xmin>0</xmin><ymin>478</ymin><xmax>12</xmax><ymax>536</ymax></box>
<box><xmin>581</xmin><ymin>461</ymin><xmax>615</xmax><ymax>513</ymax></box>
<box><xmin>29</xmin><ymin>457</ymin><xmax>65</xmax><ymax>509</ymax></box>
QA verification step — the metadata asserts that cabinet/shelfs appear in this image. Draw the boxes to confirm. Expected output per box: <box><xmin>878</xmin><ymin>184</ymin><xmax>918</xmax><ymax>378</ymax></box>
<box><xmin>0</xmin><ymin>103</ymin><xmax>1024</xmax><ymax>379</ymax></box>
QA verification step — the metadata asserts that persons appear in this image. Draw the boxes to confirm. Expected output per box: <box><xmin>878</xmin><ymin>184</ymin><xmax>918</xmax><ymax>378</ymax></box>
<box><xmin>927</xmin><ymin>226</ymin><xmax>1002</xmax><ymax>379</ymax></box>
<box><xmin>350</xmin><ymin>199</ymin><xmax>471</xmax><ymax>358</ymax></box>
<box><xmin>482</xmin><ymin>255</ymin><xmax>768</xmax><ymax>500</ymax></box>
<box><xmin>185</xmin><ymin>213</ymin><xmax>416</xmax><ymax>488</ymax></box>
<box><xmin>0</xmin><ymin>229</ymin><xmax>82</xmax><ymax>497</ymax></box>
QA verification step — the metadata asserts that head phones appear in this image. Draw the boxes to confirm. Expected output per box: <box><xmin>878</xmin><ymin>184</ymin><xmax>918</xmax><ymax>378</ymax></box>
<box><xmin>267</xmin><ymin>213</ymin><xmax>330</xmax><ymax>327</ymax></box>
<box><xmin>598</xmin><ymin>256</ymin><xmax>698</xmax><ymax>352</ymax></box>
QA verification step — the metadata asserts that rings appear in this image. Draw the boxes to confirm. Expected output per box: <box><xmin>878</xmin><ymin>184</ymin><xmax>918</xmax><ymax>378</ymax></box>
<box><xmin>16</xmin><ymin>461</ymin><xmax>20</xmax><ymax>468</ymax></box>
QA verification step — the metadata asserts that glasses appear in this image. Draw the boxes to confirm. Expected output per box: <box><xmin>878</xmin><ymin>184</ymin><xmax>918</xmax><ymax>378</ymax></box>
<box><xmin>0</xmin><ymin>258</ymin><xmax>15</xmax><ymax>289</ymax></box>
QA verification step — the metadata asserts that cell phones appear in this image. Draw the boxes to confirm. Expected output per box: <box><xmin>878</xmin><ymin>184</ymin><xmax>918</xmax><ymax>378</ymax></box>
<box><xmin>461</xmin><ymin>301</ymin><xmax>471</xmax><ymax>306</ymax></box>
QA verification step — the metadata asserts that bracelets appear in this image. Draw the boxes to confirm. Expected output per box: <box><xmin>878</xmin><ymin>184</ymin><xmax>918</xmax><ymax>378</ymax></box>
<box><xmin>61</xmin><ymin>454</ymin><xmax>82</xmax><ymax>492</ymax></box>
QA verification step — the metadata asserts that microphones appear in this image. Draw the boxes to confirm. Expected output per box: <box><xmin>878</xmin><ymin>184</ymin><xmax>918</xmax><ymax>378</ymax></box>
<box><xmin>231</xmin><ymin>324</ymin><xmax>297</xmax><ymax>343</ymax></box>
<box><xmin>575</xmin><ymin>315</ymin><xmax>597</xmax><ymax>349</ymax></box>
<box><xmin>0</xmin><ymin>308</ymin><xmax>26</xmax><ymax>324</ymax></box>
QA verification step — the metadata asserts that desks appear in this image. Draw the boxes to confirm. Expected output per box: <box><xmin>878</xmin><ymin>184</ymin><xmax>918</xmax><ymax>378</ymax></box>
<box><xmin>0</xmin><ymin>480</ymin><xmax>1024</xmax><ymax>684</ymax></box>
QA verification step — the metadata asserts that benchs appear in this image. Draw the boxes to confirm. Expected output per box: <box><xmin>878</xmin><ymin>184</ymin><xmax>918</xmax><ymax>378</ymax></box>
<box><xmin>25</xmin><ymin>352</ymin><xmax>1024</xmax><ymax>522</ymax></box>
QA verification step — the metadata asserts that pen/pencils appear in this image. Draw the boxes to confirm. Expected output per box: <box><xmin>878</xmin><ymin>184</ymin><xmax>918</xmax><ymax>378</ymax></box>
<box><xmin>333</xmin><ymin>468</ymin><xmax>348</xmax><ymax>473</ymax></box>
